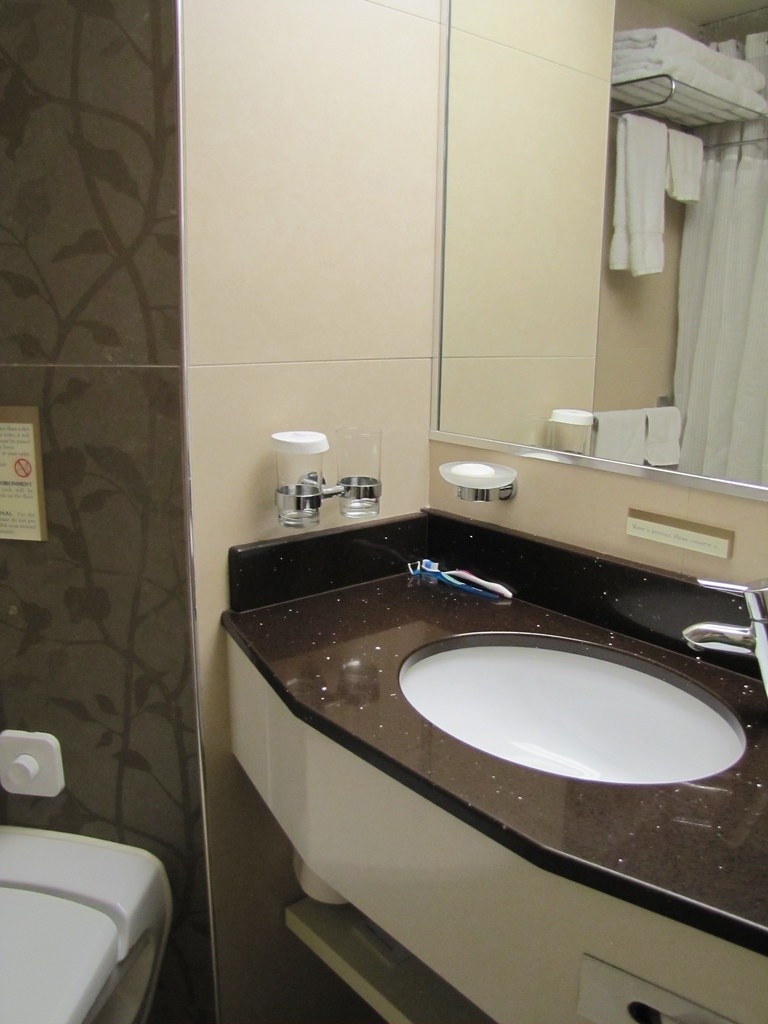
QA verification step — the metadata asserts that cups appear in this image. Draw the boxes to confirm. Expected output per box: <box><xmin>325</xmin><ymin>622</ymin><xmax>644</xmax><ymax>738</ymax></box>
<box><xmin>271</xmin><ymin>431</ymin><xmax>329</xmax><ymax>530</ymax></box>
<box><xmin>336</xmin><ymin>426</ymin><xmax>381</xmax><ymax>519</ymax></box>
<box><xmin>549</xmin><ymin>409</ymin><xmax>593</xmax><ymax>454</ymax></box>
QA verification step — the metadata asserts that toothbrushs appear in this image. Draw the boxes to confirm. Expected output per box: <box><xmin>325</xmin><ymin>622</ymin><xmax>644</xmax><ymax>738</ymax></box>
<box><xmin>407</xmin><ymin>558</ymin><xmax>515</xmax><ymax>603</ymax></box>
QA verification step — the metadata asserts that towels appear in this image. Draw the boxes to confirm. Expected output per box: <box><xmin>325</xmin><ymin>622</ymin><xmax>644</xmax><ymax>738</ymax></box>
<box><xmin>593</xmin><ymin>407</ymin><xmax>647</xmax><ymax>473</ymax></box>
<box><xmin>646</xmin><ymin>405</ymin><xmax>683</xmax><ymax>468</ymax></box>
<box><xmin>607</xmin><ymin>110</ymin><xmax>669</xmax><ymax>283</ymax></box>
<box><xmin>670</xmin><ymin>128</ymin><xmax>706</xmax><ymax>205</ymax></box>
<box><xmin>611</xmin><ymin>22</ymin><xmax>768</xmax><ymax>125</ymax></box>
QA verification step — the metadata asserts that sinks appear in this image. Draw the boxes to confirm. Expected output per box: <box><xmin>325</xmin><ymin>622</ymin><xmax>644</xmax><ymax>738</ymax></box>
<box><xmin>395</xmin><ymin>623</ymin><xmax>750</xmax><ymax>788</ymax></box>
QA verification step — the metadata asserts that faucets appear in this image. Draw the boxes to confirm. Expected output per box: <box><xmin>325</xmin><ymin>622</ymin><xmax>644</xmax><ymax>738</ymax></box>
<box><xmin>680</xmin><ymin>619</ymin><xmax>756</xmax><ymax>656</ymax></box>
